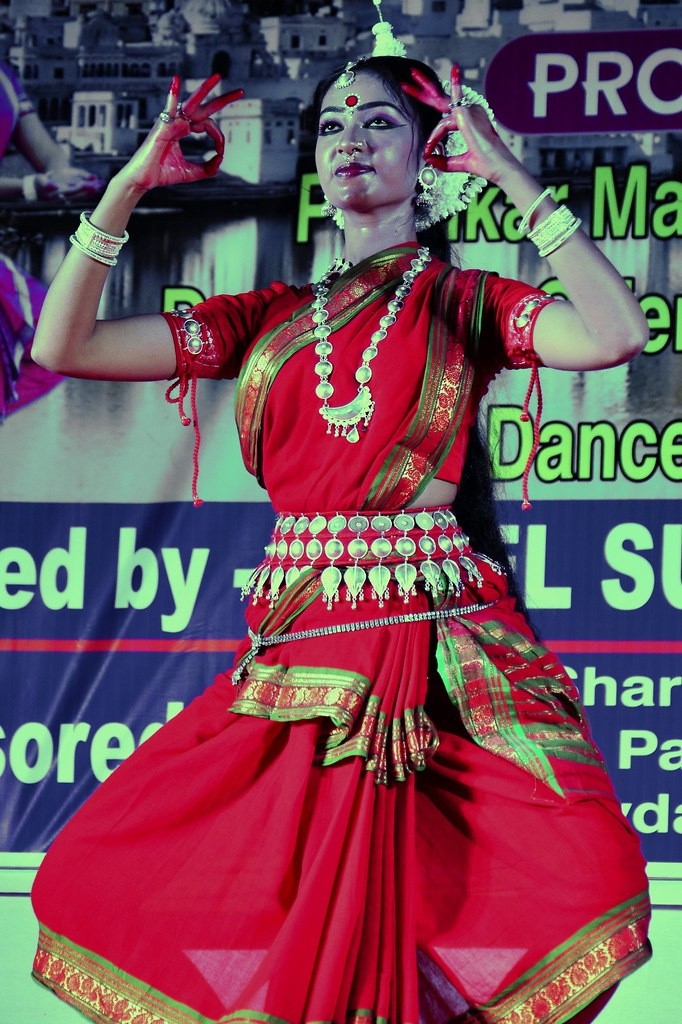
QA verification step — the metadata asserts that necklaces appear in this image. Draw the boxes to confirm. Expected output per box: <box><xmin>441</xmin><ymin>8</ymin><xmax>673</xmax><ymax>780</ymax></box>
<box><xmin>312</xmin><ymin>247</ymin><xmax>431</xmax><ymax>443</ymax></box>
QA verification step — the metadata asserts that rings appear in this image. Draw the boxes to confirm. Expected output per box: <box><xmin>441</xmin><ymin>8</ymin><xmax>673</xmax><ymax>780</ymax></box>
<box><xmin>159</xmin><ymin>113</ymin><xmax>173</xmax><ymax>123</ymax></box>
<box><xmin>185</xmin><ymin>116</ymin><xmax>192</xmax><ymax>125</ymax></box>
<box><xmin>448</xmin><ymin>102</ymin><xmax>462</xmax><ymax>109</ymax></box>
<box><xmin>177</xmin><ymin>103</ymin><xmax>185</xmax><ymax>116</ymax></box>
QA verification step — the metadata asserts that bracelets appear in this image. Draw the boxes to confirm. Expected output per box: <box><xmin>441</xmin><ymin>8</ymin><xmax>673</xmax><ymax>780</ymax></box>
<box><xmin>69</xmin><ymin>211</ymin><xmax>129</xmax><ymax>266</ymax></box>
<box><xmin>518</xmin><ymin>188</ymin><xmax>582</xmax><ymax>257</ymax></box>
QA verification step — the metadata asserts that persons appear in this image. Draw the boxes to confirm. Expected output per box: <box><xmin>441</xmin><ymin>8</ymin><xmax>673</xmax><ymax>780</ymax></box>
<box><xmin>30</xmin><ymin>56</ymin><xmax>648</xmax><ymax>1024</ymax></box>
<box><xmin>0</xmin><ymin>61</ymin><xmax>104</xmax><ymax>420</ymax></box>
